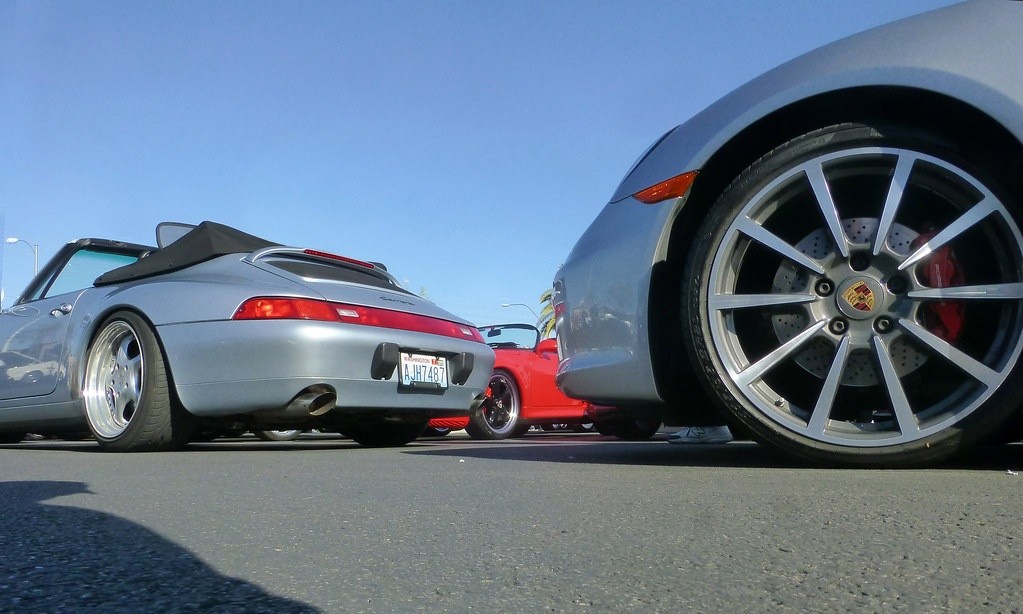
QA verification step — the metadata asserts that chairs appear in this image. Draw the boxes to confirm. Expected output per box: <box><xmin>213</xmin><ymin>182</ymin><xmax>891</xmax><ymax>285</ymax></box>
<box><xmin>497</xmin><ymin>342</ymin><xmax>516</xmax><ymax>348</ymax></box>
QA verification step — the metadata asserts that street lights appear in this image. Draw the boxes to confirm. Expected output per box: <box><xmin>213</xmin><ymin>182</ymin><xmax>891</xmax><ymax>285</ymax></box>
<box><xmin>4</xmin><ymin>236</ymin><xmax>39</xmax><ymax>277</ymax></box>
<box><xmin>502</xmin><ymin>303</ymin><xmax>546</xmax><ymax>325</ymax></box>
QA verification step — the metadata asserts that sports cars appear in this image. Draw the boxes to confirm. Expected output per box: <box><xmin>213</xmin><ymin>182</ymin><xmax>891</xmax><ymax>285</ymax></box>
<box><xmin>424</xmin><ymin>322</ymin><xmax>658</xmax><ymax>441</ymax></box>
<box><xmin>0</xmin><ymin>221</ymin><xmax>495</xmax><ymax>452</ymax></box>
<box><xmin>551</xmin><ymin>0</ymin><xmax>1023</xmax><ymax>470</ymax></box>
